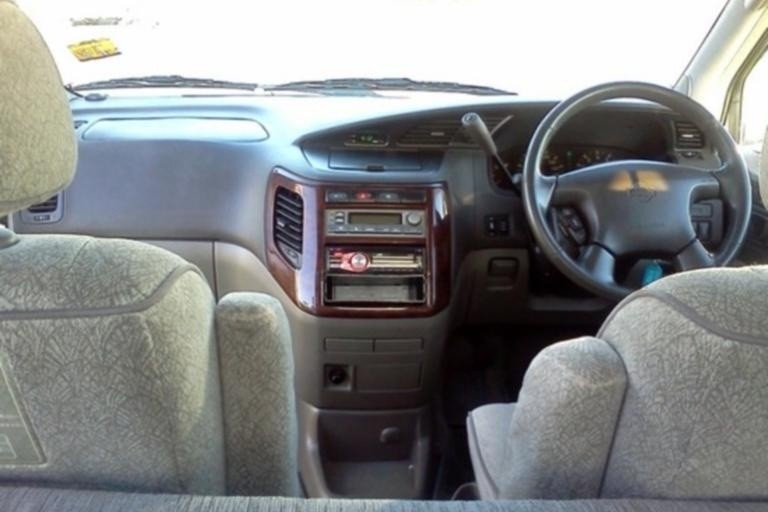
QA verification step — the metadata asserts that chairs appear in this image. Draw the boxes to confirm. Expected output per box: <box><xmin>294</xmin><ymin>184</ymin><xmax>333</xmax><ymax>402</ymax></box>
<box><xmin>0</xmin><ymin>0</ymin><xmax>304</xmax><ymax>499</ymax></box>
<box><xmin>466</xmin><ymin>263</ymin><xmax>766</xmax><ymax>510</ymax></box>
